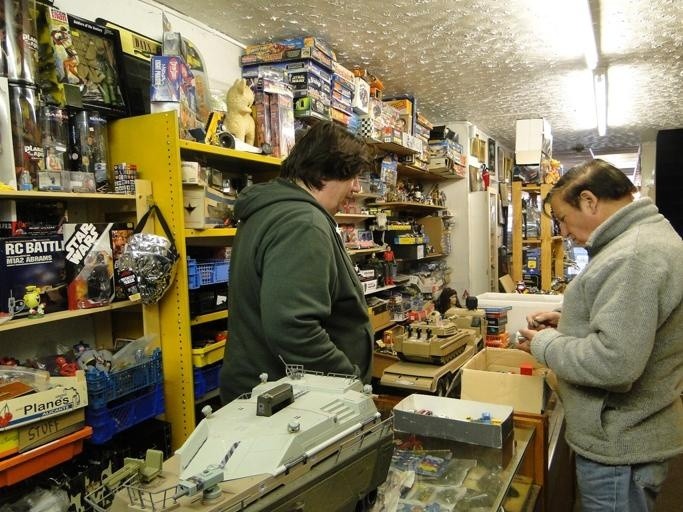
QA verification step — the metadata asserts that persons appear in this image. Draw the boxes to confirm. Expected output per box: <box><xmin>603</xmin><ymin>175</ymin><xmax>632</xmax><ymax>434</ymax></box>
<box><xmin>216</xmin><ymin>122</ymin><xmax>374</xmax><ymax>407</ymax></box>
<box><xmin>507</xmin><ymin>158</ymin><xmax>683</xmax><ymax>512</ymax></box>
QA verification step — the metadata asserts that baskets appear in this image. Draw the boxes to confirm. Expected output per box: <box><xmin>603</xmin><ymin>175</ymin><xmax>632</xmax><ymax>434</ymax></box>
<box><xmin>88</xmin><ymin>347</ymin><xmax>167</xmax><ymax>442</ymax></box>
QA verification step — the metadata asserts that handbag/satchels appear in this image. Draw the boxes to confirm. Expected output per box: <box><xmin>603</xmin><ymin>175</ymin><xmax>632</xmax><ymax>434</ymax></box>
<box><xmin>113</xmin><ymin>205</ymin><xmax>180</xmax><ymax>307</ymax></box>
